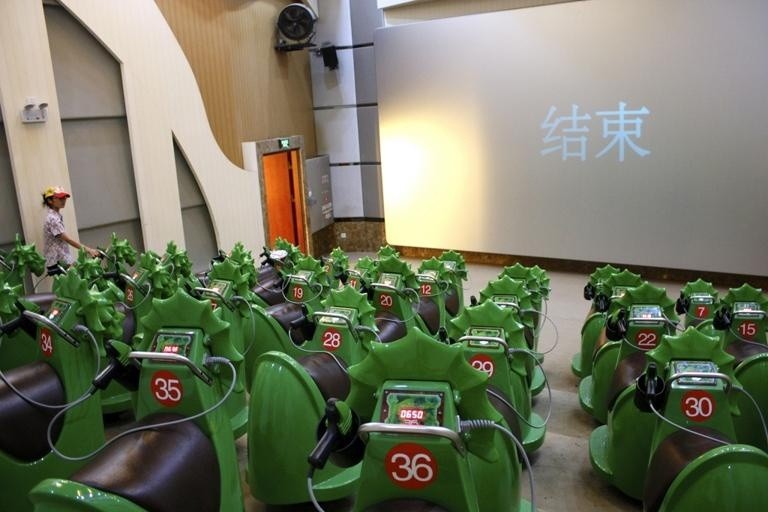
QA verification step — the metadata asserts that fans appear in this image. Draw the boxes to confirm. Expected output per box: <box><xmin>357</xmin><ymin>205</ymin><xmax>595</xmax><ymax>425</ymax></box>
<box><xmin>276</xmin><ymin>3</ymin><xmax>317</xmax><ymax>50</ymax></box>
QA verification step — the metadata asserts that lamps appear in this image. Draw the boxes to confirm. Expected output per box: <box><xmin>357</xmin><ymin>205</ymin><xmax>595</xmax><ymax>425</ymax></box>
<box><xmin>20</xmin><ymin>97</ymin><xmax>49</xmax><ymax>123</ymax></box>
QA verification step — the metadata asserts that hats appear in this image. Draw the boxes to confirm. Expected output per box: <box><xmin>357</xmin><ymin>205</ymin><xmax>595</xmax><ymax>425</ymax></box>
<box><xmin>44</xmin><ymin>186</ymin><xmax>70</xmax><ymax>199</ymax></box>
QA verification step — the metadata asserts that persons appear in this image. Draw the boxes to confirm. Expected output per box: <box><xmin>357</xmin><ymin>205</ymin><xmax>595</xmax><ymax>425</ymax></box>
<box><xmin>41</xmin><ymin>185</ymin><xmax>101</xmax><ymax>277</ymax></box>
